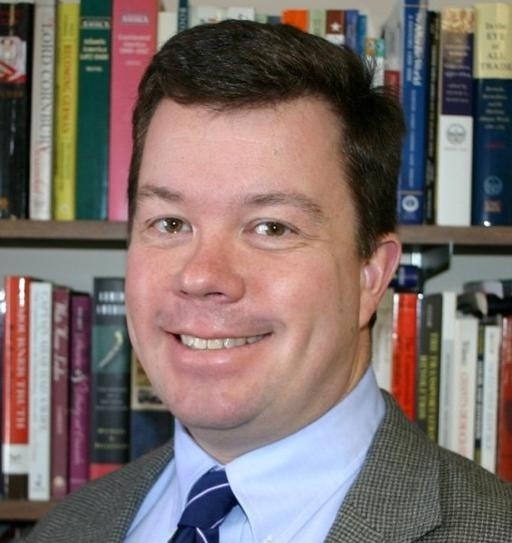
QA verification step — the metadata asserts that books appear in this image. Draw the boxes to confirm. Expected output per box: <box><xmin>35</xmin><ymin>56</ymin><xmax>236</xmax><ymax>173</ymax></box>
<box><xmin>367</xmin><ymin>280</ymin><xmax>512</xmax><ymax>482</ymax></box>
<box><xmin>1</xmin><ymin>275</ymin><xmax>174</xmax><ymax>503</ymax></box>
<box><xmin>0</xmin><ymin>0</ymin><xmax>512</xmax><ymax>228</ymax></box>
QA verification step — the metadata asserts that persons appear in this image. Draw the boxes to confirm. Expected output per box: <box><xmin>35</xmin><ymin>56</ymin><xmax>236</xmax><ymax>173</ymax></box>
<box><xmin>17</xmin><ymin>18</ymin><xmax>512</xmax><ymax>542</ymax></box>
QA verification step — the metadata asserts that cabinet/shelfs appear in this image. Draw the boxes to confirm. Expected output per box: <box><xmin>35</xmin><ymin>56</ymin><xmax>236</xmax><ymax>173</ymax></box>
<box><xmin>0</xmin><ymin>221</ymin><xmax>511</xmax><ymax>527</ymax></box>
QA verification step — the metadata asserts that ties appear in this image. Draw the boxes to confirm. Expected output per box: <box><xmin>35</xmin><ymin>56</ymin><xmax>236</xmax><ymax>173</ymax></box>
<box><xmin>168</xmin><ymin>470</ymin><xmax>238</xmax><ymax>543</ymax></box>
